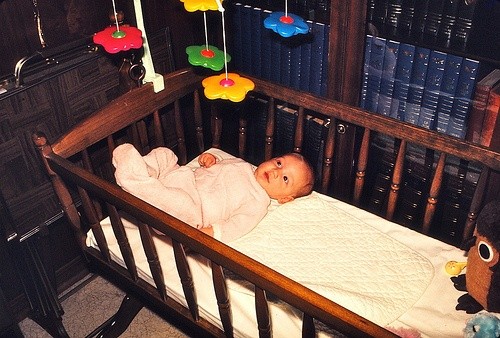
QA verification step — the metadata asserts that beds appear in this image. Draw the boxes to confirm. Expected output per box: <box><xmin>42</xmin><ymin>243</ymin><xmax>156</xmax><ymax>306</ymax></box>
<box><xmin>32</xmin><ymin>59</ymin><xmax>500</xmax><ymax>338</ymax></box>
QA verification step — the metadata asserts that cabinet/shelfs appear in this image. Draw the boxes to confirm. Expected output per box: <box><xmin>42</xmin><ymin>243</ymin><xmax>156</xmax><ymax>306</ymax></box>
<box><xmin>0</xmin><ymin>0</ymin><xmax>500</xmax><ymax>323</ymax></box>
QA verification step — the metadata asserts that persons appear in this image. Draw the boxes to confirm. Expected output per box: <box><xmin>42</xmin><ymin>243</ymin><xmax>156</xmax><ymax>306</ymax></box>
<box><xmin>111</xmin><ymin>142</ymin><xmax>318</xmax><ymax>252</ymax></box>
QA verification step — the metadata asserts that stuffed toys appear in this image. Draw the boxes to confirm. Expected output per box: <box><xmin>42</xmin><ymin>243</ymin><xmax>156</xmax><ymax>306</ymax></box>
<box><xmin>462</xmin><ymin>312</ymin><xmax>500</xmax><ymax>338</ymax></box>
<box><xmin>450</xmin><ymin>199</ymin><xmax>500</xmax><ymax>315</ymax></box>
